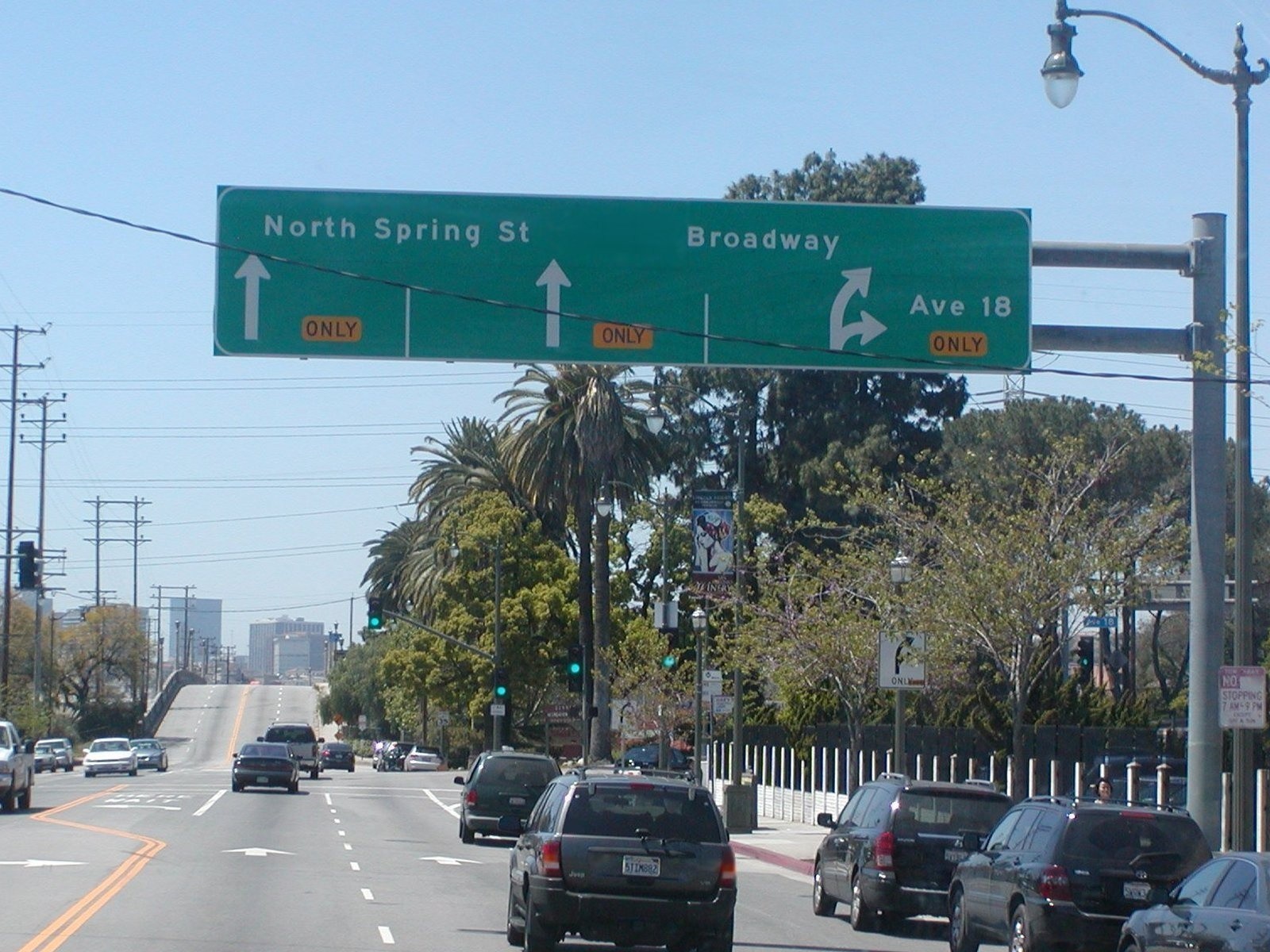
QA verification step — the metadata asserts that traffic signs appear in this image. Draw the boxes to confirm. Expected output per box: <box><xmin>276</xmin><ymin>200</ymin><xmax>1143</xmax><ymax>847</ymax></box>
<box><xmin>212</xmin><ymin>181</ymin><xmax>1037</xmax><ymax>383</ymax></box>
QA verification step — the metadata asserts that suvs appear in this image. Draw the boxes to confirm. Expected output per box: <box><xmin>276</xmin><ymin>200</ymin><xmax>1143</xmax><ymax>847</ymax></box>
<box><xmin>507</xmin><ymin>765</ymin><xmax>738</xmax><ymax>952</ymax></box>
<box><xmin>811</xmin><ymin>772</ymin><xmax>1212</xmax><ymax>952</ymax></box>
<box><xmin>258</xmin><ymin>722</ymin><xmax>325</xmax><ymax>780</ymax></box>
<box><xmin>455</xmin><ymin>748</ymin><xmax>562</xmax><ymax>842</ymax></box>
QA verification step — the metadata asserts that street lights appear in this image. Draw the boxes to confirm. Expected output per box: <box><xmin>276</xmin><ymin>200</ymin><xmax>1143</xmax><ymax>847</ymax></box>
<box><xmin>653</xmin><ymin>0</ymin><xmax>1269</xmax><ymax>849</ymax></box>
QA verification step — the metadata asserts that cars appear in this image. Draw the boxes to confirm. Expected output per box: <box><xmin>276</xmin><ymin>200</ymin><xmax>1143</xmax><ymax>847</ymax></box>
<box><xmin>232</xmin><ymin>742</ymin><xmax>300</xmax><ymax>794</ymax></box>
<box><xmin>1117</xmin><ymin>851</ymin><xmax>1270</xmax><ymax>952</ymax></box>
<box><xmin>319</xmin><ymin>740</ymin><xmax>442</xmax><ymax>773</ymax></box>
<box><xmin>34</xmin><ymin>737</ymin><xmax>169</xmax><ymax>782</ymax></box>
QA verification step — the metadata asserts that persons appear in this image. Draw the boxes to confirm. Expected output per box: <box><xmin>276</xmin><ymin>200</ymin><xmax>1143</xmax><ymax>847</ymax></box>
<box><xmin>1093</xmin><ymin>777</ymin><xmax>1115</xmax><ymax>806</ymax></box>
<box><xmin>376</xmin><ymin>740</ymin><xmax>398</xmax><ymax>772</ymax></box>
<box><xmin>685</xmin><ymin>758</ymin><xmax>703</xmax><ymax>787</ymax></box>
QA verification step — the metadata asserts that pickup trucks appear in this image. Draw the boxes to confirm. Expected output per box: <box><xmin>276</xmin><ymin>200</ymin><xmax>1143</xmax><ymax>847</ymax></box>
<box><xmin>0</xmin><ymin>721</ymin><xmax>37</xmax><ymax>811</ymax></box>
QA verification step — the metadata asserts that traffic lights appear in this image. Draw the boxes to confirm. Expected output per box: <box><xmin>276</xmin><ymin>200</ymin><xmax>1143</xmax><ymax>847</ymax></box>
<box><xmin>367</xmin><ymin>599</ymin><xmax>382</xmax><ymax>631</ymax></box>
<box><xmin>493</xmin><ymin>667</ymin><xmax>510</xmax><ymax>699</ymax></box>
<box><xmin>568</xmin><ymin>644</ymin><xmax>583</xmax><ymax>694</ymax></box>
